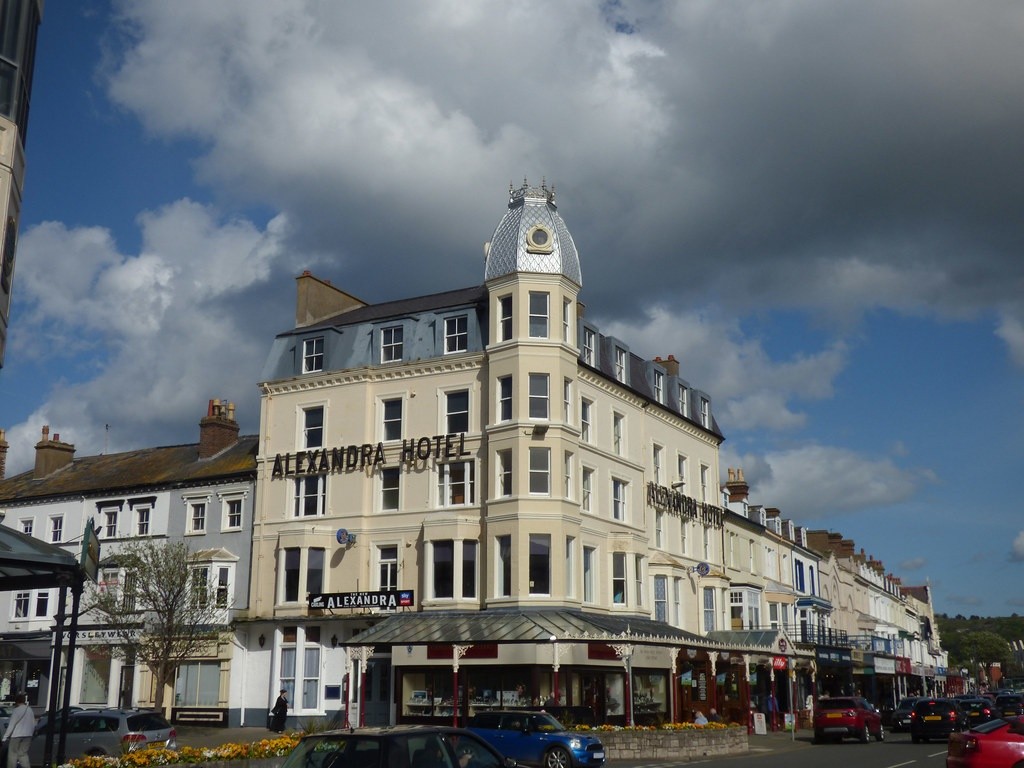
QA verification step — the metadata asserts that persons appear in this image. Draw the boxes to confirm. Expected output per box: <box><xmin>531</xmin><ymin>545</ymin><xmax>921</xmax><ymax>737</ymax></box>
<box><xmin>544</xmin><ymin>691</ymin><xmax>561</xmax><ymax>706</ymax></box>
<box><xmin>695</xmin><ymin>711</ymin><xmax>708</xmax><ymax>724</ymax></box>
<box><xmin>0</xmin><ymin>673</ymin><xmax>11</xmax><ymax>702</ymax></box>
<box><xmin>768</xmin><ymin>689</ymin><xmax>782</xmax><ymax>730</ymax></box>
<box><xmin>2</xmin><ymin>695</ymin><xmax>36</xmax><ymax>768</ymax></box>
<box><xmin>437</xmin><ymin>734</ymin><xmax>473</xmax><ymax>768</ymax></box>
<box><xmin>824</xmin><ymin>691</ymin><xmax>830</xmax><ymax>699</ymax></box>
<box><xmin>970</xmin><ymin>684</ymin><xmax>976</xmax><ymax>695</ymax></box>
<box><xmin>271</xmin><ymin>689</ymin><xmax>289</xmax><ymax>734</ymax></box>
<box><xmin>517</xmin><ymin>685</ymin><xmax>525</xmax><ymax>699</ymax></box>
<box><xmin>707</xmin><ymin>708</ymin><xmax>719</xmax><ymax>723</ymax></box>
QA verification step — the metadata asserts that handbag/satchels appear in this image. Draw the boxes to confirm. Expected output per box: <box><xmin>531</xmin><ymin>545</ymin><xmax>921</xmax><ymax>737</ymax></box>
<box><xmin>2</xmin><ymin>737</ymin><xmax>9</xmax><ymax>750</ymax></box>
<box><xmin>271</xmin><ymin>707</ymin><xmax>280</xmax><ymax>715</ymax></box>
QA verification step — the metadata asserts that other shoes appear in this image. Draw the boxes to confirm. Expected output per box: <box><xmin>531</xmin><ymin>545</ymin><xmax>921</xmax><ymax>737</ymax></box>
<box><xmin>278</xmin><ymin>731</ymin><xmax>281</xmax><ymax>733</ymax></box>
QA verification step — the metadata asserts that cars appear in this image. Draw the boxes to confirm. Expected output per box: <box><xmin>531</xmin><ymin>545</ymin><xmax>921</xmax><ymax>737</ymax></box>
<box><xmin>910</xmin><ymin>698</ymin><xmax>971</xmax><ymax>744</ymax></box>
<box><xmin>278</xmin><ymin>725</ymin><xmax>529</xmax><ymax>768</ymax></box>
<box><xmin>17</xmin><ymin>708</ymin><xmax>176</xmax><ymax>768</ymax></box>
<box><xmin>464</xmin><ymin>711</ymin><xmax>605</xmax><ymax>768</ymax></box>
<box><xmin>813</xmin><ymin>697</ymin><xmax>884</xmax><ymax>743</ymax></box>
<box><xmin>953</xmin><ymin>689</ymin><xmax>1024</xmax><ymax>728</ymax></box>
<box><xmin>946</xmin><ymin>716</ymin><xmax>1024</xmax><ymax>768</ymax></box>
<box><xmin>890</xmin><ymin>697</ymin><xmax>927</xmax><ymax>732</ymax></box>
